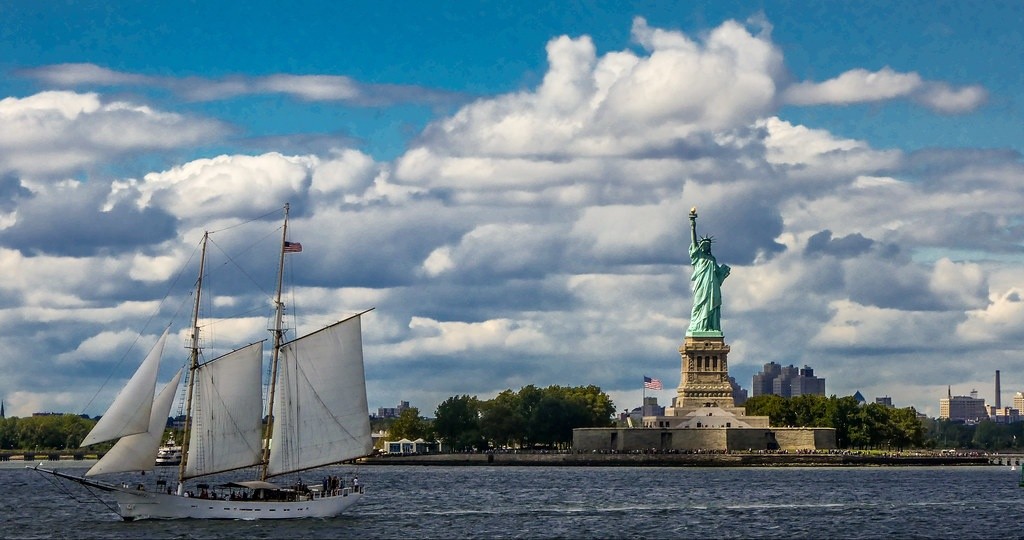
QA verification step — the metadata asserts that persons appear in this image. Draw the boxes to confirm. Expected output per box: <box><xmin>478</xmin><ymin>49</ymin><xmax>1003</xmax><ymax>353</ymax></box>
<box><xmin>744</xmin><ymin>446</ymin><xmax>789</xmax><ymax>456</ymax></box>
<box><xmin>684</xmin><ymin>207</ymin><xmax>731</xmax><ymax>338</ymax></box>
<box><xmin>166</xmin><ymin>485</ymin><xmax>261</xmax><ymax>501</ymax></box>
<box><xmin>795</xmin><ymin>446</ymin><xmax>867</xmax><ymax>457</ymax></box>
<box><xmin>322</xmin><ymin>474</ymin><xmax>360</xmax><ymax>494</ymax></box>
<box><xmin>375</xmin><ymin>446</ymin><xmax>740</xmax><ymax>456</ymax></box>
<box><xmin>882</xmin><ymin>449</ymin><xmax>902</xmax><ymax>460</ymax></box>
<box><xmin>908</xmin><ymin>449</ymin><xmax>1002</xmax><ymax>457</ymax></box>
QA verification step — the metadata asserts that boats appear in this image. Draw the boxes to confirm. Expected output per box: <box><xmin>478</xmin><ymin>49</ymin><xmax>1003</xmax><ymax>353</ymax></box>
<box><xmin>154</xmin><ymin>429</ymin><xmax>183</xmax><ymax>467</ymax></box>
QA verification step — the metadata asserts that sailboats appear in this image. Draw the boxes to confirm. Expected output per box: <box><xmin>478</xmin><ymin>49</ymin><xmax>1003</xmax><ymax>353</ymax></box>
<box><xmin>24</xmin><ymin>201</ymin><xmax>377</xmax><ymax>522</ymax></box>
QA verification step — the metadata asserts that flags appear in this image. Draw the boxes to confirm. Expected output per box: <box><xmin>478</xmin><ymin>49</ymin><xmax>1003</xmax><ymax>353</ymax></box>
<box><xmin>283</xmin><ymin>241</ymin><xmax>303</xmax><ymax>253</ymax></box>
<box><xmin>644</xmin><ymin>374</ymin><xmax>663</xmax><ymax>391</ymax></box>
